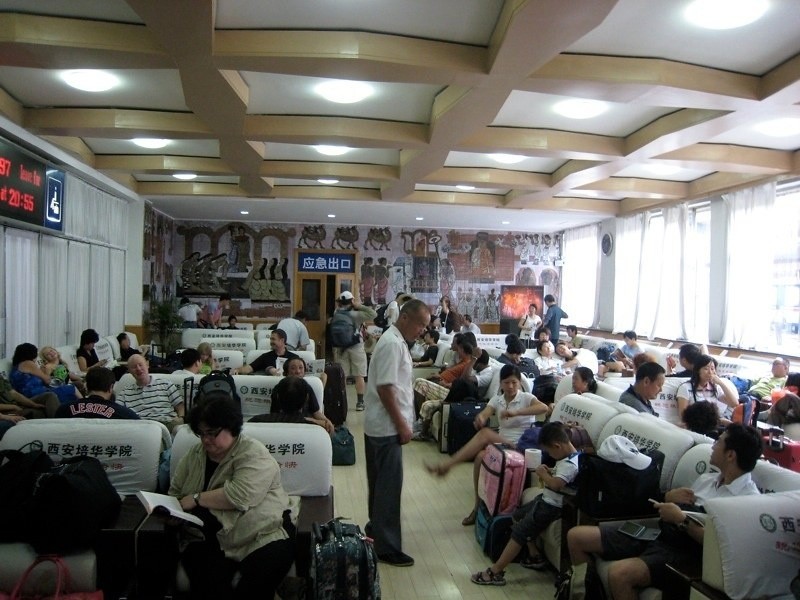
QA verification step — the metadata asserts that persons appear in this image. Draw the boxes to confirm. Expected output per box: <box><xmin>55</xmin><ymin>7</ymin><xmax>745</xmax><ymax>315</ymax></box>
<box><xmin>0</xmin><ymin>290</ymin><xmax>800</xmax><ymax>600</ymax></box>
<box><xmin>619</xmin><ymin>361</ymin><xmax>666</xmax><ymax>419</ymax></box>
<box><xmin>232</xmin><ymin>328</ymin><xmax>304</xmax><ymax>376</ymax></box>
<box><xmin>167</xmin><ymin>387</ymin><xmax>297</xmax><ymax>600</ymax></box>
<box><xmin>115</xmin><ymin>354</ymin><xmax>186</xmax><ymax>435</ymax></box>
<box><xmin>567</xmin><ymin>420</ymin><xmax>764</xmax><ymax>600</ymax></box>
<box><xmin>54</xmin><ymin>365</ymin><xmax>144</xmax><ymax>420</ymax></box>
<box><xmin>359</xmin><ymin>300</ymin><xmax>431</xmax><ymax>567</ymax></box>
<box><xmin>542</xmin><ymin>294</ymin><xmax>569</xmax><ymax>344</ymax></box>
<box><xmin>424</xmin><ymin>364</ymin><xmax>549</xmax><ymax>526</ymax></box>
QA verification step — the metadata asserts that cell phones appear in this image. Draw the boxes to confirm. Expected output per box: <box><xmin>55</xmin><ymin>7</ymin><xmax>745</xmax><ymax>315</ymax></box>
<box><xmin>618</xmin><ymin>520</ymin><xmax>645</xmax><ymax>537</ymax></box>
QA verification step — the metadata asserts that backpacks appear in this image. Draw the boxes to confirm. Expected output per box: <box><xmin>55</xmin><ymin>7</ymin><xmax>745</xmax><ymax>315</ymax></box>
<box><xmin>193</xmin><ymin>371</ymin><xmax>241</xmax><ymax>408</ymax></box>
<box><xmin>374</xmin><ymin>301</ymin><xmax>393</xmax><ymax>327</ymax></box>
<box><xmin>326</xmin><ymin>306</ymin><xmax>360</xmax><ymax>348</ymax></box>
<box><xmin>554</xmin><ymin>562</ymin><xmax>608</xmax><ymax>600</ymax></box>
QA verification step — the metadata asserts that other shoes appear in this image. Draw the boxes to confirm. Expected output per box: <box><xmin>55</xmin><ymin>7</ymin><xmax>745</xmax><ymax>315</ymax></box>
<box><xmin>356</xmin><ymin>403</ymin><xmax>363</xmax><ymax>411</ymax></box>
<box><xmin>377</xmin><ymin>550</ymin><xmax>414</xmax><ymax>567</ymax></box>
<box><xmin>426</xmin><ymin>465</ymin><xmax>449</xmax><ymax>477</ymax></box>
<box><xmin>410</xmin><ymin>422</ymin><xmax>430</xmax><ymax>442</ymax></box>
<box><xmin>462</xmin><ymin>511</ymin><xmax>476</xmax><ymax>525</ymax></box>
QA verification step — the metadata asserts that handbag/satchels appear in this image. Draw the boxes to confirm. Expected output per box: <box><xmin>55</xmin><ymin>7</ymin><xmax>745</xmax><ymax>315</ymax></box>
<box><xmin>0</xmin><ymin>449</ymin><xmax>123</xmax><ymax>555</ymax></box>
<box><xmin>574</xmin><ymin>449</ymin><xmax>666</xmax><ymax>517</ymax></box>
<box><xmin>516</xmin><ymin>421</ymin><xmax>596</xmax><ymax>468</ymax></box>
<box><xmin>0</xmin><ymin>553</ymin><xmax>104</xmax><ymax>600</ymax></box>
<box><xmin>330</xmin><ymin>427</ymin><xmax>355</xmax><ymax>466</ymax></box>
<box><xmin>475</xmin><ymin>443</ymin><xmax>528</xmax><ymax>563</ymax></box>
<box><xmin>729</xmin><ymin>376</ymin><xmax>753</xmax><ymax>394</ymax></box>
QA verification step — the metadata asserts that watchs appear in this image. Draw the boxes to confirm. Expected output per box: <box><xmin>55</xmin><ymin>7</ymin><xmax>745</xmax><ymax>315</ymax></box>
<box><xmin>193</xmin><ymin>492</ymin><xmax>202</xmax><ymax>507</ymax></box>
<box><xmin>678</xmin><ymin>514</ymin><xmax>690</xmax><ymax>532</ymax></box>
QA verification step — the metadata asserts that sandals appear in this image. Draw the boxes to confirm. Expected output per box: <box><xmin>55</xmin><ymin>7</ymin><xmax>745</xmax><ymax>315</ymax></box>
<box><xmin>470</xmin><ymin>568</ymin><xmax>506</xmax><ymax>586</ymax></box>
<box><xmin>520</xmin><ymin>553</ymin><xmax>545</xmax><ymax>568</ymax></box>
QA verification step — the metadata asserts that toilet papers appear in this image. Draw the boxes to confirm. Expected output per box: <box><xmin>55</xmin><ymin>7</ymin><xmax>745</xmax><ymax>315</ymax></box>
<box><xmin>524</xmin><ymin>448</ymin><xmax>542</xmax><ymax>469</ymax></box>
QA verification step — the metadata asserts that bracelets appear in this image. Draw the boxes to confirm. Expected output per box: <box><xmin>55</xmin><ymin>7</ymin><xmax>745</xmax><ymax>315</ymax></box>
<box><xmin>472</xmin><ymin>356</ymin><xmax>477</xmax><ymax>360</ymax></box>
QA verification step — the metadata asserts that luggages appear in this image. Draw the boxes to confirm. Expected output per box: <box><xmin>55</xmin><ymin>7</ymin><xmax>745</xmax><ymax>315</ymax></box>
<box><xmin>324</xmin><ymin>361</ymin><xmax>348</xmax><ymax>425</ymax></box>
<box><xmin>761</xmin><ymin>427</ymin><xmax>800</xmax><ymax>473</ymax></box>
<box><xmin>446</xmin><ymin>397</ymin><xmax>490</xmax><ymax>462</ymax></box>
<box><xmin>312</xmin><ymin>517</ymin><xmax>382</xmax><ymax>600</ymax></box>
<box><xmin>148</xmin><ymin>344</ymin><xmax>175</xmax><ymax>374</ymax></box>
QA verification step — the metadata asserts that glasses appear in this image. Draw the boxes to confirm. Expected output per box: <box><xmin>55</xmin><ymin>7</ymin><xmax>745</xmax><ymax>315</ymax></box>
<box><xmin>195</xmin><ymin>422</ymin><xmax>225</xmax><ymax>438</ymax></box>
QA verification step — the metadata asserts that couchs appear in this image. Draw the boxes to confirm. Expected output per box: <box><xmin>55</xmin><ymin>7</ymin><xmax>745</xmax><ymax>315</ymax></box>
<box><xmin>0</xmin><ymin>327</ymin><xmax>334</xmax><ymax>600</ymax></box>
<box><xmin>413</xmin><ymin>334</ymin><xmax>800</xmax><ymax>600</ymax></box>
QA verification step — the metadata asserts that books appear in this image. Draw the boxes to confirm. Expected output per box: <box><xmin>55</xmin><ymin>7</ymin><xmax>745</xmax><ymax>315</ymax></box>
<box><xmin>135</xmin><ymin>490</ymin><xmax>204</xmax><ymax>528</ymax></box>
<box><xmin>609</xmin><ymin>348</ymin><xmax>627</xmax><ymax>362</ymax></box>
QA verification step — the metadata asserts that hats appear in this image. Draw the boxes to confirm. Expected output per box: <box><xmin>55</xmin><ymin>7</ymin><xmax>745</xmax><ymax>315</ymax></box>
<box><xmin>597</xmin><ymin>435</ymin><xmax>652</xmax><ymax>470</ymax></box>
<box><xmin>338</xmin><ymin>291</ymin><xmax>353</xmax><ymax>300</ymax></box>
